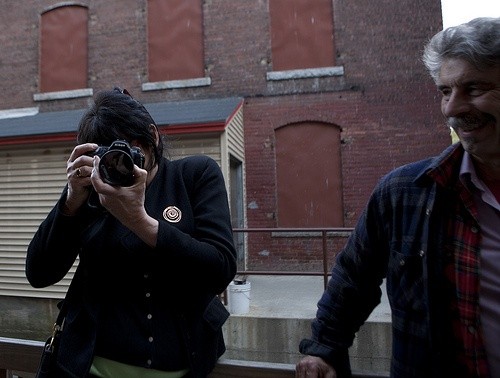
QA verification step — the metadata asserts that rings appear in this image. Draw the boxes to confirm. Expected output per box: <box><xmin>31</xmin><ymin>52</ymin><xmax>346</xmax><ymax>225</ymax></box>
<box><xmin>76</xmin><ymin>168</ymin><xmax>82</xmax><ymax>176</ymax></box>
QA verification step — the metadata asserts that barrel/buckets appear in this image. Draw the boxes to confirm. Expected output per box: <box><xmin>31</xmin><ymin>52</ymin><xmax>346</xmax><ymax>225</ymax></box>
<box><xmin>226</xmin><ymin>281</ymin><xmax>251</xmax><ymax>314</ymax></box>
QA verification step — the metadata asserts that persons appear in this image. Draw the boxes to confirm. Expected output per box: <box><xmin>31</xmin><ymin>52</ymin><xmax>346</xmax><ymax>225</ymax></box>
<box><xmin>296</xmin><ymin>16</ymin><xmax>500</xmax><ymax>378</ymax></box>
<box><xmin>26</xmin><ymin>90</ymin><xmax>236</xmax><ymax>378</ymax></box>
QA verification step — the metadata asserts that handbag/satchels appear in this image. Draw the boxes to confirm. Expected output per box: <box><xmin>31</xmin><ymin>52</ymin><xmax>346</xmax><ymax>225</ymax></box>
<box><xmin>34</xmin><ymin>325</ymin><xmax>62</xmax><ymax>378</ymax></box>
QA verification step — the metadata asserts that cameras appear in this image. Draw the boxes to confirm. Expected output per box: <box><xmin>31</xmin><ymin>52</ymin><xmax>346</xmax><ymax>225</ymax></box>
<box><xmin>86</xmin><ymin>139</ymin><xmax>145</xmax><ymax>187</ymax></box>
